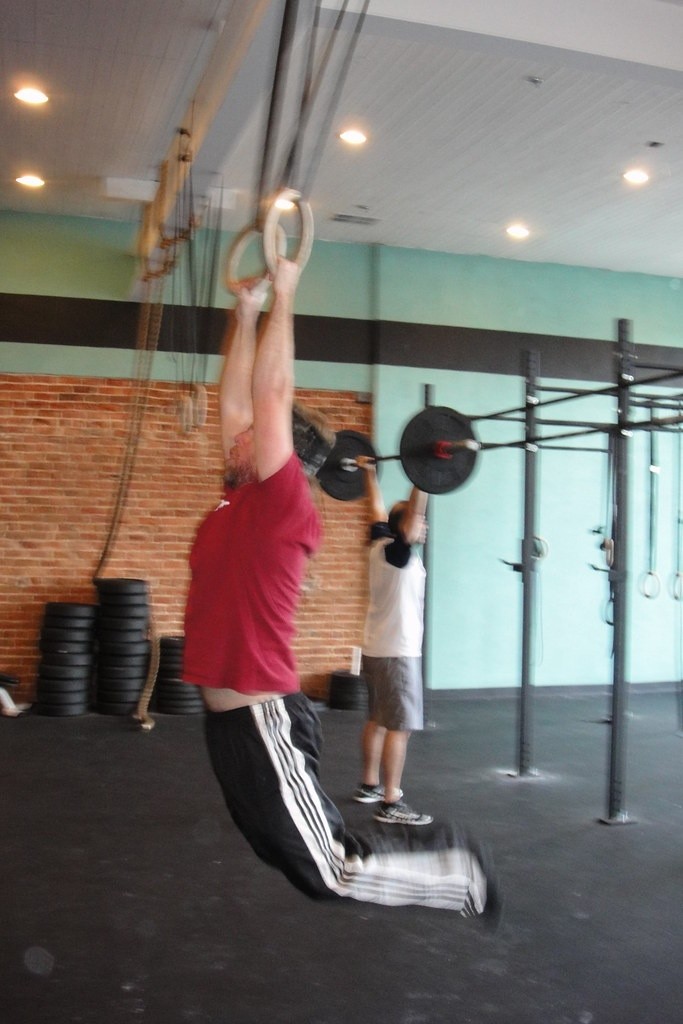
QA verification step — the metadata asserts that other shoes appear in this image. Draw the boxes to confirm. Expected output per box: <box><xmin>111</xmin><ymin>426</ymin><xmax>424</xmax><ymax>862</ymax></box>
<box><xmin>373</xmin><ymin>797</ymin><xmax>434</xmax><ymax>825</ymax></box>
<box><xmin>351</xmin><ymin>784</ymin><xmax>403</xmax><ymax>803</ymax></box>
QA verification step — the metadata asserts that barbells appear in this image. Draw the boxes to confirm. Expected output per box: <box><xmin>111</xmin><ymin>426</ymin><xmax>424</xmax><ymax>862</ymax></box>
<box><xmin>312</xmin><ymin>405</ymin><xmax>479</xmax><ymax>502</ymax></box>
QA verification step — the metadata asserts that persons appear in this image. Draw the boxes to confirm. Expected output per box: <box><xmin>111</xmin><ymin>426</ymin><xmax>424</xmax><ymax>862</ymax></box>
<box><xmin>352</xmin><ymin>454</ymin><xmax>437</xmax><ymax>826</ymax></box>
<box><xmin>184</xmin><ymin>255</ymin><xmax>502</xmax><ymax>928</ymax></box>
